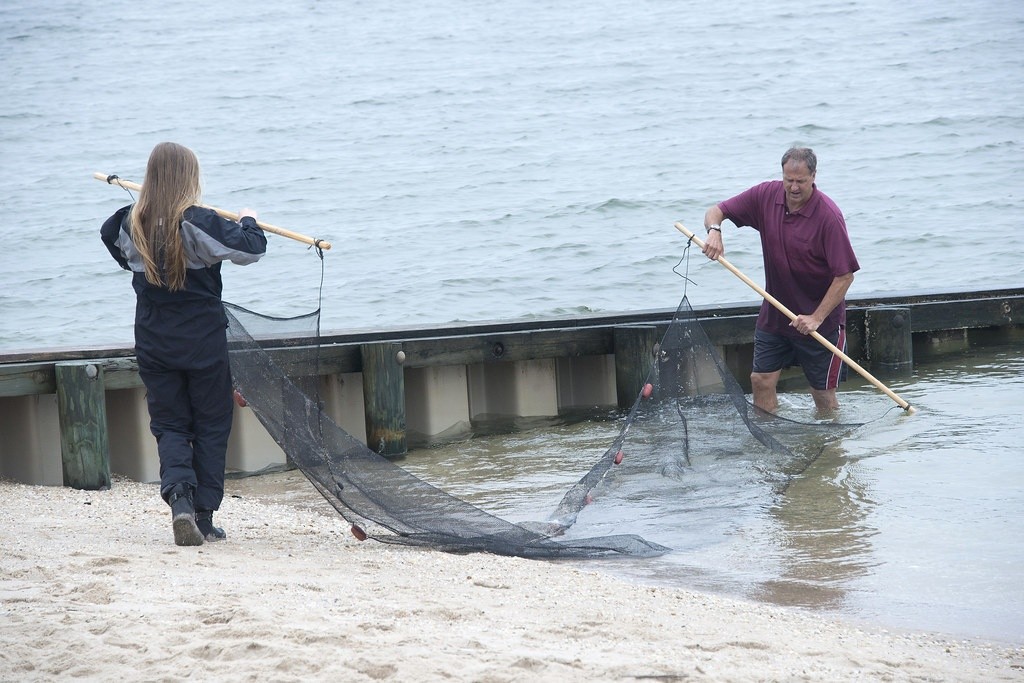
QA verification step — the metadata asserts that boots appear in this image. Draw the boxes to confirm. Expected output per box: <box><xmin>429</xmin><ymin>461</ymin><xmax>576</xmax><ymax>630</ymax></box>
<box><xmin>166</xmin><ymin>482</ymin><xmax>205</xmax><ymax>546</ymax></box>
<box><xmin>194</xmin><ymin>507</ymin><xmax>226</xmax><ymax>542</ymax></box>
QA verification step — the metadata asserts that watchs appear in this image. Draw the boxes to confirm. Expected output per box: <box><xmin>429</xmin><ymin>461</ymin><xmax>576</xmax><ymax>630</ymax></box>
<box><xmin>707</xmin><ymin>224</ymin><xmax>722</xmax><ymax>234</ymax></box>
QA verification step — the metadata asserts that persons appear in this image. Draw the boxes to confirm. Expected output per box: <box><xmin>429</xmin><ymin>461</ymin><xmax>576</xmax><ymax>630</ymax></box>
<box><xmin>701</xmin><ymin>147</ymin><xmax>860</xmax><ymax>414</ymax></box>
<box><xmin>100</xmin><ymin>142</ymin><xmax>267</xmax><ymax>546</ymax></box>
